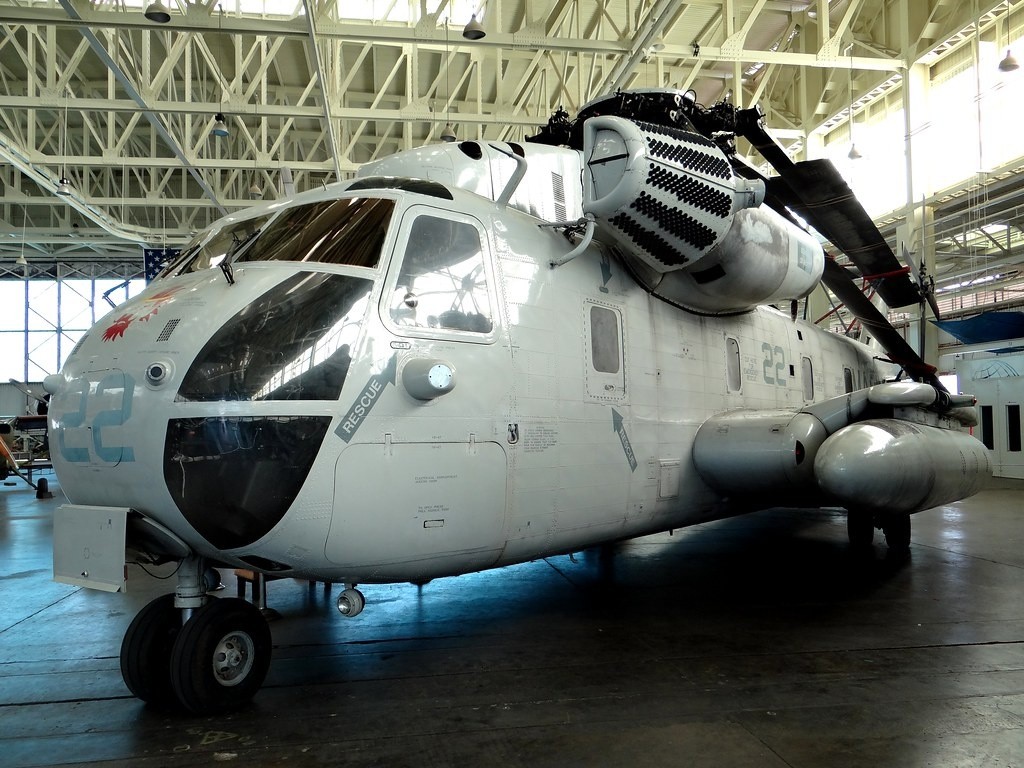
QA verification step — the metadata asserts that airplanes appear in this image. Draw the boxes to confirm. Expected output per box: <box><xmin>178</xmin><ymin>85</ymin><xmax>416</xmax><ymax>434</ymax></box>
<box><xmin>6</xmin><ymin>5</ymin><xmax>997</xmax><ymax>717</ymax></box>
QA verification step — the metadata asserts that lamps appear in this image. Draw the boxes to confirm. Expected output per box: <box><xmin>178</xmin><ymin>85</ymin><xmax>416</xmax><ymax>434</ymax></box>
<box><xmin>15</xmin><ymin>190</ymin><xmax>29</xmax><ymax>265</ymax></box>
<box><xmin>439</xmin><ymin>16</ymin><xmax>457</xmax><ymax>142</ymax></box>
<box><xmin>847</xmin><ymin>45</ymin><xmax>863</xmax><ymax>160</ymax></box>
<box><xmin>144</xmin><ymin>0</ymin><xmax>172</xmax><ymax>23</ymax></box>
<box><xmin>462</xmin><ymin>14</ymin><xmax>487</xmax><ymax>40</ymax></box>
<box><xmin>56</xmin><ymin>85</ymin><xmax>72</xmax><ymax>196</ymax></box>
<box><xmin>998</xmin><ymin>0</ymin><xmax>1020</xmax><ymax>72</ymax></box>
<box><xmin>210</xmin><ymin>12</ymin><xmax>230</xmax><ymax>137</ymax></box>
<box><xmin>249</xmin><ymin>94</ymin><xmax>263</xmax><ymax>197</ymax></box>
<box><xmin>651</xmin><ymin>37</ymin><xmax>665</xmax><ymax>51</ymax></box>
